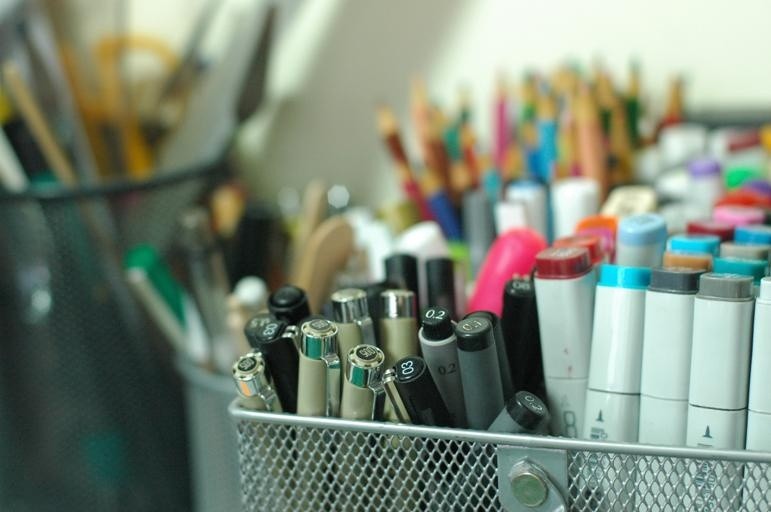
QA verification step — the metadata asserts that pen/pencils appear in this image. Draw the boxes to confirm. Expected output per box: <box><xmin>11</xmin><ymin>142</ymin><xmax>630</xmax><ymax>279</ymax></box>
<box><xmin>232</xmin><ymin>213</ymin><xmax>771</xmax><ymax>512</ymax></box>
<box><xmin>126</xmin><ymin>178</ymin><xmax>355</xmax><ymax>372</ymax></box>
<box><xmin>503</xmin><ymin>119</ymin><xmax>771</xmax><ymax>213</ymax></box>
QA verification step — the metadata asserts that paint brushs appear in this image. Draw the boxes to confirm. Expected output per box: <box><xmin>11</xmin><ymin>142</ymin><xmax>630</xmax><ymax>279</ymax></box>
<box><xmin>380</xmin><ymin>63</ymin><xmax>687</xmax><ymax>233</ymax></box>
